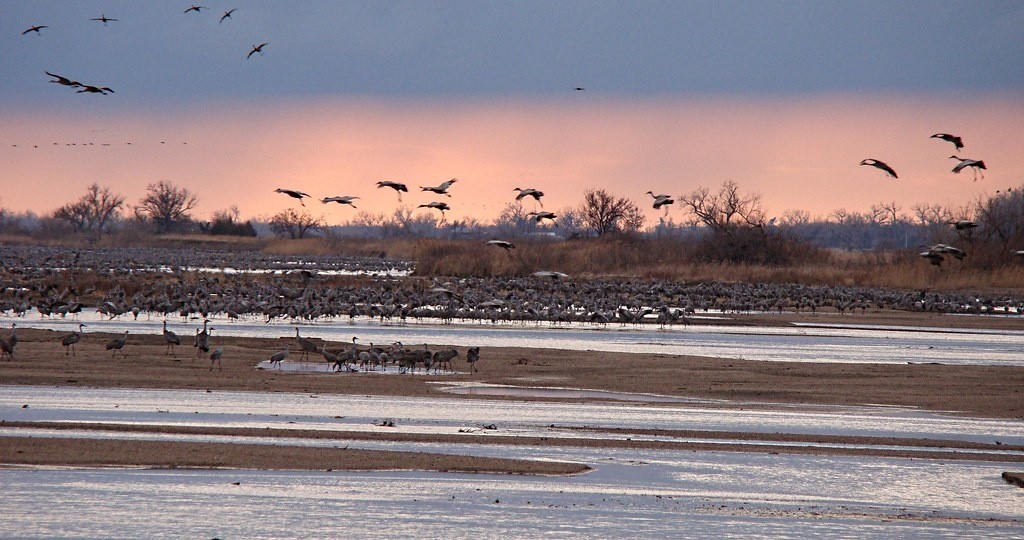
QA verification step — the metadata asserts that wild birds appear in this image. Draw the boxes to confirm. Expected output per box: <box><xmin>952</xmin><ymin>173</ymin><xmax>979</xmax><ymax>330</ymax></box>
<box><xmin>513</xmin><ymin>188</ymin><xmax>544</xmax><ymax>207</ymax></box>
<box><xmin>930</xmin><ymin>133</ymin><xmax>964</xmax><ymax>149</ymax></box>
<box><xmin>418</xmin><ymin>202</ymin><xmax>450</xmax><ymax>216</ymax></box>
<box><xmin>573</xmin><ymin>88</ymin><xmax>585</xmax><ymax>91</ymax></box>
<box><xmin>0</xmin><ymin>240</ymin><xmax>1024</xmax><ymax>389</ymax></box>
<box><xmin>13</xmin><ymin>141</ymin><xmax>187</xmax><ymax>148</ymax></box>
<box><xmin>184</xmin><ymin>4</ymin><xmax>268</xmax><ymax>58</ymax></box>
<box><xmin>529</xmin><ymin>211</ymin><xmax>558</xmax><ymax>226</ymax></box>
<box><xmin>45</xmin><ymin>71</ymin><xmax>114</xmax><ymax>95</ymax></box>
<box><xmin>90</xmin><ymin>14</ymin><xmax>118</xmax><ymax>22</ymax></box>
<box><xmin>319</xmin><ymin>196</ymin><xmax>360</xmax><ymax>208</ymax></box>
<box><xmin>274</xmin><ymin>189</ymin><xmax>310</xmax><ymax>207</ymax></box>
<box><xmin>861</xmin><ymin>158</ymin><xmax>897</xmax><ymax>178</ymax></box>
<box><xmin>949</xmin><ymin>155</ymin><xmax>987</xmax><ymax>182</ymax></box>
<box><xmin>419</xmin><ymin>178</ymin><xmax>457</xmax><ymax>197</ymax></box>
<box><xmin>646</xmin><ymin>191</ymin><xmax>674</xmax><ymax>209</ymax></box>
<box><xmin>375</xmin><ymin>181</ymin><xmax>408</xmax><ymax>202</ymax></box>
<box><xmin>945</xmin><ymin>217</ymin><xmax>978</xmax><ymax>240</ymax></box>
<box><xmin>22</xmin><ymin>25</ymin><xmax>47</xmax><ymax>37</ymax></box>
